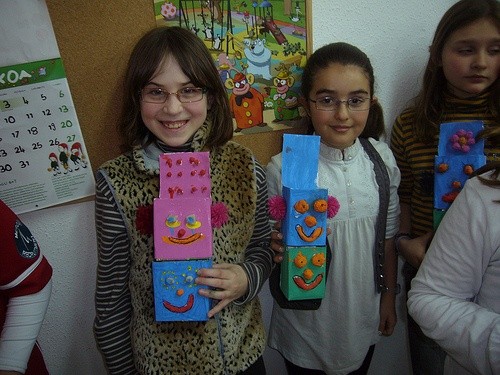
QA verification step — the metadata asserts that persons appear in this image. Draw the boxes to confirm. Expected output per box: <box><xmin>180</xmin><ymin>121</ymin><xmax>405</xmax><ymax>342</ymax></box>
<box><xmin>92</xmin><ymin>25</ymin><xmax>276</xmax><ymax>375</ymax></box>
<box><xmin>390</xmin><ymin>0</ymin><xmax>500</xmax><ymax>375</ymax></box>
<box><xmin>0</xmin><ymin>200</ymin><xmax>53</xmax><ymax>375</ymax></box>
<box><xmin>408</xmin><ymin>164</ymin><xmax>500</xmax><ymax>375</ymax></box>
<box><xmin>266</xmin><ymin>42</ymin><xmax>402</xmax><ymax>375</ymax></box>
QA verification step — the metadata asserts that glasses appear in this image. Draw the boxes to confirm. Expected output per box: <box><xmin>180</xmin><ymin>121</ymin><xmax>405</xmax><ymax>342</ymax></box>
<box><xmin>311</xmin><ymin>96</ymin><xmax>369</xmax><ymax>111</ymax></box>
<box><xmin>139</xmin><ymin>87</ymin><xmax>207</xmax><ymax>103</ymax></box>
